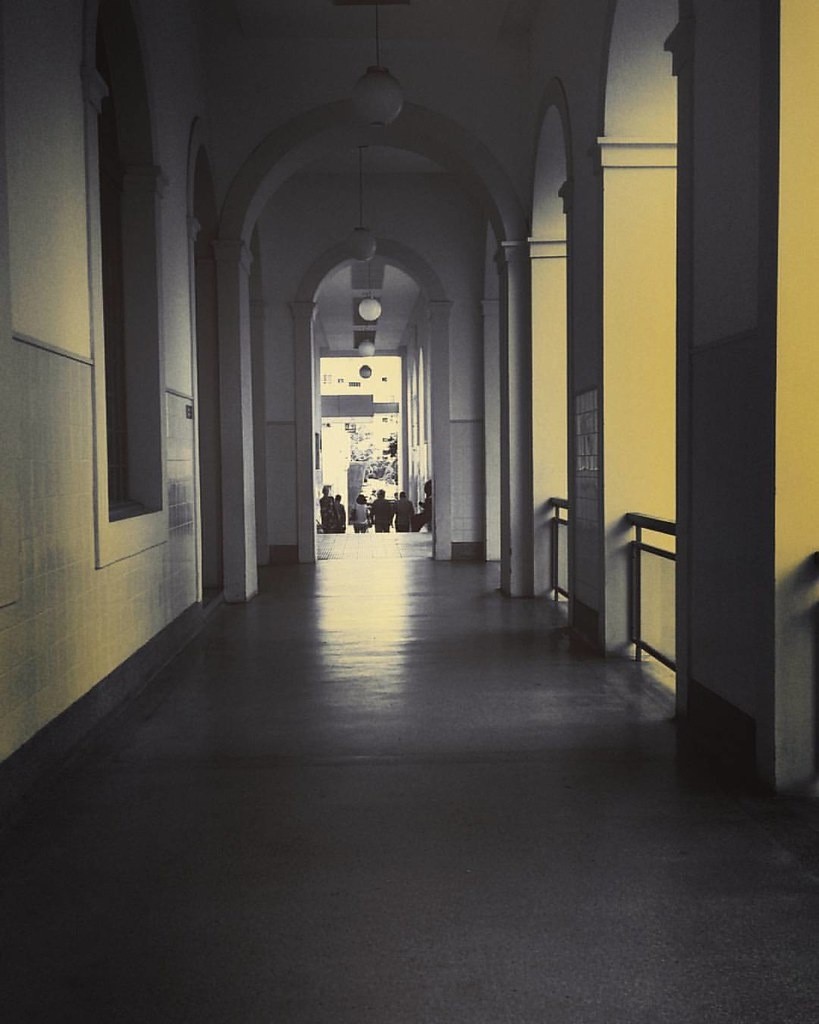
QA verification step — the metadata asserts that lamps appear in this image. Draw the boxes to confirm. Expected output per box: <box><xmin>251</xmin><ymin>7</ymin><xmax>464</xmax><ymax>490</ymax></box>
<box><xmin>359</xmin><ymin>365</ymin><xmax>372</xmax><ymax>378</ymax></box>
<box><xmin>346</xmin><ymin>146</ymin><xmax>377</xmax><ymax>261</ymax></box>
<box><xmin>351</xmin><ymin>0</ymin><xmax>405</xmax><ymax>127</ymax></box>
<box><xmin>358</xmin><ymin>262</ymin><xmax>381</xmax><ymax>321</ymax></box>
<box><xmin>358</xmin><ymin>322</ymin><xmax>375</xmax><ymax>358</ymax></box>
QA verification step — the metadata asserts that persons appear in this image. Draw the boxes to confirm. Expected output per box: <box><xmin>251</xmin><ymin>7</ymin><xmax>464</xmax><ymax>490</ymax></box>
<box><xmin>350</xmin><ymin>494</ymin><xmax>372</xmax><ymax>533</ymax></box>
<box><xmin>391</xmin><ymin>479</ymin><xmax>432</xmax><ymax>532</ymax></box>
<box><xmin>320</xmin><ymin>486</ymin><xmax>346</xmax><ymax>534</ymax></box>
<box><xmin>369</xmin><ymin>488</ymin><xmax>392</xmax><ymax>532</ymax></box>
